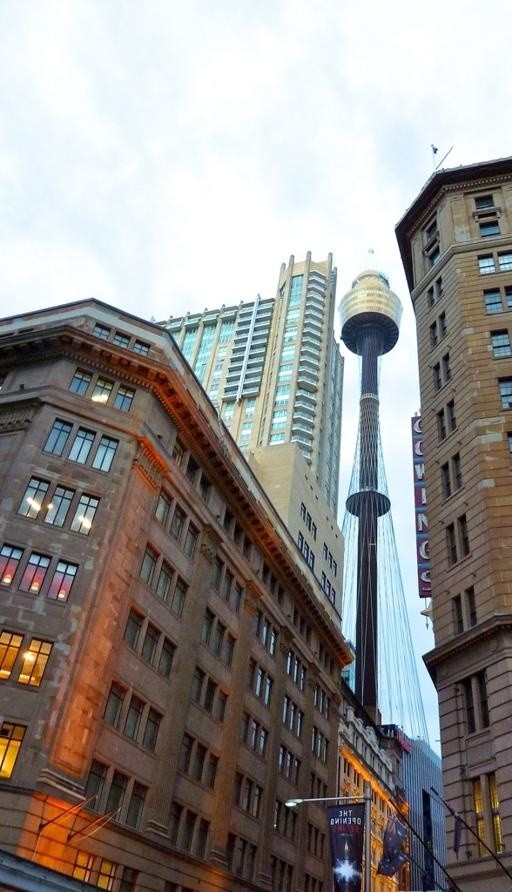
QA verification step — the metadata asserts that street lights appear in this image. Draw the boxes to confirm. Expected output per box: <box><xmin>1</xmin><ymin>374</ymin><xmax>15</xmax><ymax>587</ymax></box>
<box><xmin>283</xmin><ymin>781</ymin><xmax>372</xmax><ymax>892</ymax></box>
